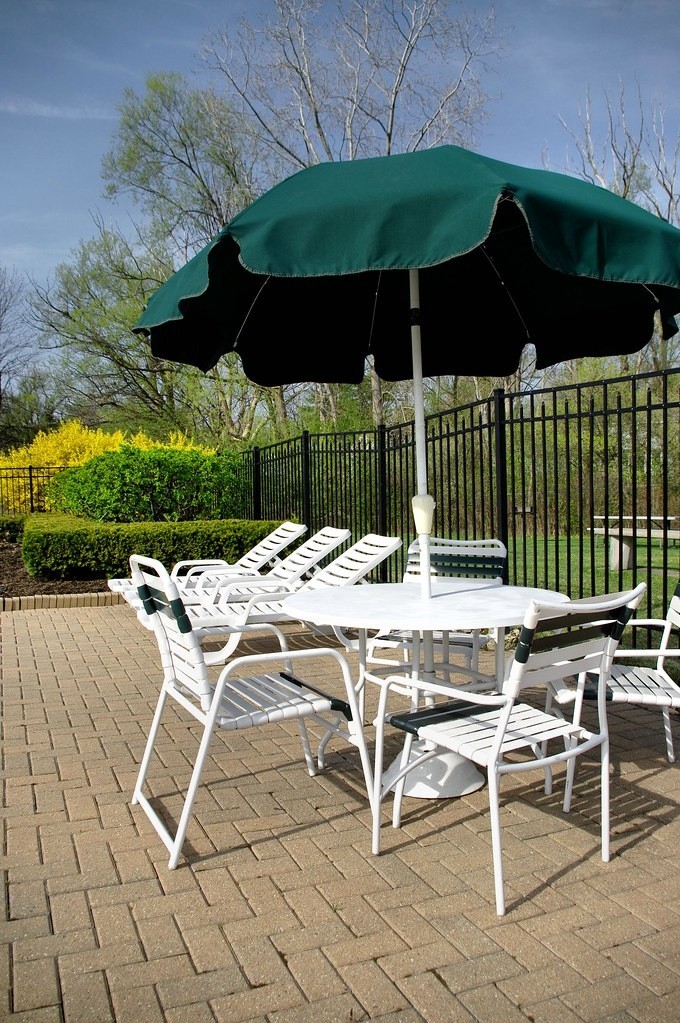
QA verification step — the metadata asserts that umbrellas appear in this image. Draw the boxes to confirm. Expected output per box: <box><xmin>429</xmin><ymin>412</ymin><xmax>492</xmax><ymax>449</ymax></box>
<box><xmin>131</xmin><ymin>145</ymin><xmax>680</xmax><ymax>754</ymax></box>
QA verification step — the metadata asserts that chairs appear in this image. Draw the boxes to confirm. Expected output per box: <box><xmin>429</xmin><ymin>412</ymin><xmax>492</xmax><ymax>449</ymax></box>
<box><xmin>103</xmin><ymin>520</ymin><xmax>680</xmax><ymax>916</ymax></box>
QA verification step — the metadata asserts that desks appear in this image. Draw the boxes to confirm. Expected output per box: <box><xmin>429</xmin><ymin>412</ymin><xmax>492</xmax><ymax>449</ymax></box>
<box><xmin>285</xmin><ymin>581</ymin><xmax>572</xmax><ymax>799</ymax></box>
